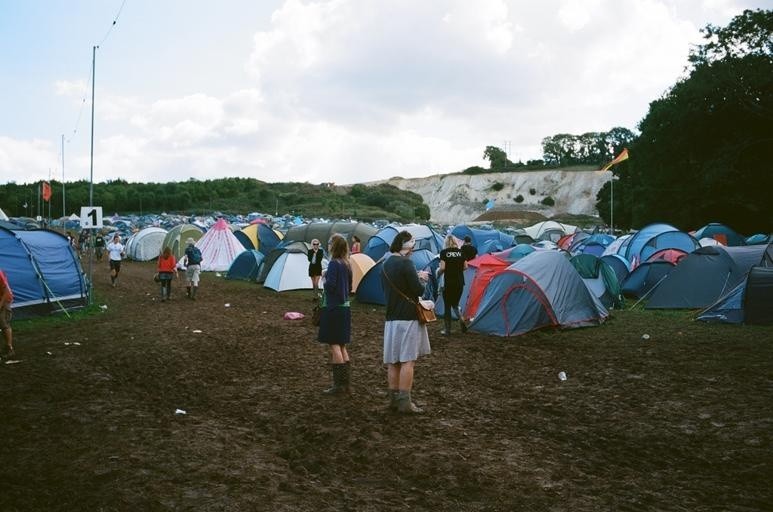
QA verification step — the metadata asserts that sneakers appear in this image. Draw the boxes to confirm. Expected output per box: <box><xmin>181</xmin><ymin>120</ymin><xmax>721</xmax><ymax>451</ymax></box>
<box><xmin>0</xmin><ymin>350</ymin><xmax>15</xmax><ymax>359</ymax></box>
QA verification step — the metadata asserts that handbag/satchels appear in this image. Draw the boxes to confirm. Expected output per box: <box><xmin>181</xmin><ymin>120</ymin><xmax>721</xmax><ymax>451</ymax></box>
<box><xmin>415</xmin><ymin>299</ymin><xmax>437</xmax><ymax>324</ymax></box>
<box><xmin>312</xmin><ymin>306</ymin><xmax>323</xmax><ymax>326</ymax></box>
<box><xmin>153</xmin><ymin>272</ymin><xmax>161</xmax><ymax>282</ymax></box>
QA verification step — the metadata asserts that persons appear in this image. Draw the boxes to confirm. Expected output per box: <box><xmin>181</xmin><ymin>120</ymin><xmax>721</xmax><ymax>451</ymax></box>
<box><xmin>0</xmin><ymin>269</ymin><xmax>15</xmax><ymax>360</ymax></box>
<box><xmin>311</xmin><ymin>233</ymin><xmax>353</xmax><ymax>394</ymax></box>
<box><xmin>381</xmin><ymin>231</ymin><xmax>437</xmax><ymax>414</ymax></box>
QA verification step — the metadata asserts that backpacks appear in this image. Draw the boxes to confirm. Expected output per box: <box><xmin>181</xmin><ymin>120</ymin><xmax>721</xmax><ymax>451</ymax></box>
<box><xmin>190</xmin><ymin>248</ymin><xmax>202</xmax><ymax>263</ymax></box>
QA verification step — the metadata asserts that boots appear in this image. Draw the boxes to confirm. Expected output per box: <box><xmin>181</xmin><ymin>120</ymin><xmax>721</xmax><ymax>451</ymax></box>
<box><xmin>313</xmin><ymin>288</ymin><xmax>319</xmax><ymax>299</ymax></box>
<box><xmin>390</xmin><ymin>390</ymin><xmax>424</xmax><ymax>414</ymax></box>
<box><xmin>183</xmin><ymin>286</ymin><xmax>198</xmax><ymax>300</ymax></box>
<box><xmin>460</xmin><ymin>318</ymin><xmax>468</xmax><ymax>332</ymax></box>
<box><xmin>323</xmin><ymin>361</ymin><xmax>352</xmax><ymax>399</ymax></box>
<box><xmin>111</xmin><ymin>275</ymin><xmax>116</xmax><ymax>288</ymax></box>
<box><xmin>441</xmin><ymin>318</ymin><xmax>452</xmax><ymax>336</ymax></box>
<box><xmin>159</xmin><ymin>286</ymin><xmax>172</xmax><ymax>302</ymax></box>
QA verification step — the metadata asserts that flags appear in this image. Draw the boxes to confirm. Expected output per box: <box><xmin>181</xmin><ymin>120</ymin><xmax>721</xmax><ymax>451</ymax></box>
<box><xmin>42</xmin><ymin>181</ymin><xmax>52</xmax><ymax>202</ymax></box>
<box><xmin>598</xmin><ymin>148</ymin><xmax>629</xmax><ymax>177</ymax></box>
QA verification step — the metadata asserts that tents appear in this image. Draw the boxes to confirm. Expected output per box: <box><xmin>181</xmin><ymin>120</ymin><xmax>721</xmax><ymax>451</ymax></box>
<box><xmin>1</xmin><ymin>212</ymin><xmax>773</xmax><ymax>341</ymax></box>
